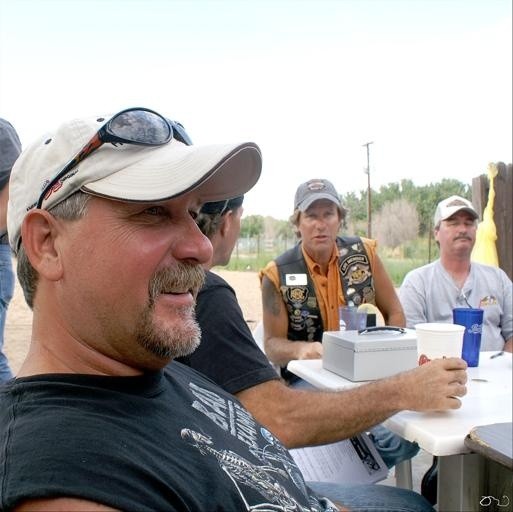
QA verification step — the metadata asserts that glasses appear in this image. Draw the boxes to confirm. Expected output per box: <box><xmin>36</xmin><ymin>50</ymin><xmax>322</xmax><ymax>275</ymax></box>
<box><xmin>38</xmin><ymin>106</ymin><xmax>194</xmax><ymax>224</ymax></box>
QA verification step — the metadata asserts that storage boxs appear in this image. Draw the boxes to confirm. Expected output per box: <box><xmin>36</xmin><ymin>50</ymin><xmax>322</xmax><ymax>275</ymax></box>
<box><xmin>323</xmin><ymin>326</ymin><xmax>419</xmax><ymax>382</ymax></box>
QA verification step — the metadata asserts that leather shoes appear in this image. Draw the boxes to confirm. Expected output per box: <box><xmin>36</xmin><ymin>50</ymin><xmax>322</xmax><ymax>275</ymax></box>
<box><xmin>420</xmin><ymin>454</ymin><xmax>439</xmax><ymax>507</ymax></box>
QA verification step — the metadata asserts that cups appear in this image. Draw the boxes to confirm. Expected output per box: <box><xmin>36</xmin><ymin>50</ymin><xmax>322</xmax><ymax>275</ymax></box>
<box><xmin>415</xmin><ymin>323</ymin><xmax>465</xmax><ymax>369</ymax></box>
<box><xmin>454</xmin><ymin>308</ymin><xmax>483</xmax><ymax>368</ymax></box>
<box><xmin>337</xmin><ymin>306</ymin><xmax>365</xmax><ymax>330</ymax></box>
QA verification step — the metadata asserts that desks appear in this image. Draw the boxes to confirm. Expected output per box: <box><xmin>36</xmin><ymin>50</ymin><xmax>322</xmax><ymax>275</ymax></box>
<box><xmin>287</xmin><ymin>350</ymin><xmax>513</xmax><ymax>512</ymax></box>
<box><xmin>463</xmin><ymin>422</ymin><xmax>513</xmax><ymax>512</ymax></box>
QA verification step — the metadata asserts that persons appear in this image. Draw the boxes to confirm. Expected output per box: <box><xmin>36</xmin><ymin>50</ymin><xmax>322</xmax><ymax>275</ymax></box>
<box><xmin>258</xmin><ymin>178</ymin><xmax>421</xmax><ymax>470</ymax></box>
<box><xmin>400</xmin><ymin>195</ymin><xmax>513</xmax><ymax>506</ymax></box>
<box><xmin>0</xmin><ymin>107</ymin><xmax>347</xmax><ymax>512</ymax></box>
<box><xmin>0</xmin><ymin>117</ymin><xmax>23</xmax><ymax>384</ymax></box>
<box><xmin>174</xmin><ymin>194</ymin><xmax>468</xmax><ymax>512</ymax></box>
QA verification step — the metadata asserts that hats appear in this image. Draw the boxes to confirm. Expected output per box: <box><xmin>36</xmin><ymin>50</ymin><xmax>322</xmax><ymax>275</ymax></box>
<box><xmin>433</xmin><ymin>195</ymin><xmax>479</xmax><ymax>231</ymax></box>
<box><xmin>293</xmin><ymin>177</ymin><xmax>346</xmax><ymax>218</ymax></box>
<box><xmin>6</xmin><ymin>102</ymin><xmax>264</xmax><ymax>256</ymax></box>
